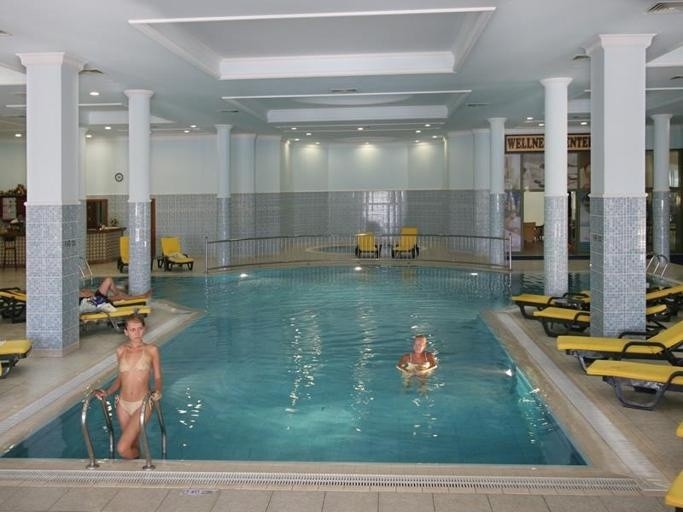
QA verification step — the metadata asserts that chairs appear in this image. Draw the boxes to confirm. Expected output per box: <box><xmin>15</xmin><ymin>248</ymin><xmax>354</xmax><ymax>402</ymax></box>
<box><xmin>0</xmin><ymin>340</ymin><xmax>31</xmax><ymax>379</ymax></box>
<box><xmin>79</xmin><ymin>299</ymin><xmax>150</xmax><ymax>334</ymax></box>
<box><xmin>355</xmin><ymin>232</ymin><xmax>380</xmax><ymax>258</ymax></box>
<box><xmin>510</xmin><ymin>283</ymin><xmax>683</xmax><ymax>512</ymax></box>
<box><xmin>0</xmin><ymin>287</ymin><xmax>26</xmax><ymax>324</ymax></box>
<box><xmin>393</xmin><ymin>228</ymin><xmax>418</xmax><ymax>259</ymax></box>
<box><xmin>117</xmin><ymin>236</ymin><xmax>130</xmax><ymax>273</ymax></box>
<box><xmin>157</xmin><ymin>238</ymin><xmax>194</xmax><ymax>271</ymax></box>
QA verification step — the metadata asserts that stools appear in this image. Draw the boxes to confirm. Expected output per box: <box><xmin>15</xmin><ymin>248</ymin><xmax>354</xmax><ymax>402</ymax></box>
<box><xmin>4</xmin><ymin>234</ymin><xmax>18</xmax><ymax>271</ymax></box>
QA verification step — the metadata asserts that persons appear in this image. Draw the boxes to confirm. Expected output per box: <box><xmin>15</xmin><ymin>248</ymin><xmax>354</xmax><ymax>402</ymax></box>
<box><xmin>393</xmin><ymin>334</ymin><xmax>440</xmax><ymax>397</ymax></box>
<box><xmin>94</xmin><ymin>311</ymin><xmax>163</xmax><ymax>460</ymax></box>
<box><xmin>503</xmin><ymin>210</ymin><xmax>520</xmax><ymax>234</ymax></box>
<box><xmin>78</xmin><ymin>275</ymin><xmax>154</xmax><ymax>305</ymax></box>
<box><xmin>6</xmin><ymin>212</ymin><xmax>25</xmax><ymax>230</ymax></box>
